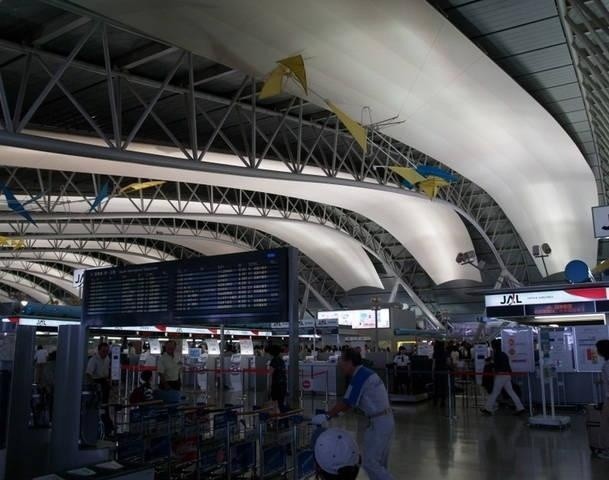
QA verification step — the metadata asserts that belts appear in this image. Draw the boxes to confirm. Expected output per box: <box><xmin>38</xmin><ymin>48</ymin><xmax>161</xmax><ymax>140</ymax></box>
<box><xmin>367</xmin><ymin>409</ymin><xmax>391</xmax><ymax>420</ymax></box>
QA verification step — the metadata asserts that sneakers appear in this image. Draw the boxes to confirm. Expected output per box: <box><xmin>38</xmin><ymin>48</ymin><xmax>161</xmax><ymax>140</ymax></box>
<box><xmin>479</xmin><ymin>408</ymin><xmax>492</xmax><ymax>415</ymax></box>
<box><xmin>512</xmin><ymin>409</ymin><xmax>525</xmax><ymax>416</ymax></box>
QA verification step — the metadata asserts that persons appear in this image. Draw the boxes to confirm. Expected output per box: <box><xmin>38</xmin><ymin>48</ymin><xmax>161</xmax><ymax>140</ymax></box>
<box><xmin>86</xmin><ymin>342</ymin><xmax>119</xmax><ymax>436</ymax></box>
<box><xmin>140</xmin><ymin>369</ymin><xmax>154</xmax><ymax>415</ymax></box>
<box><xmin>480</xmin><ymin>341</ymin><xmax>528</xmax><ymax>417</ymax></box>
<box><xmin>121</xmin><ymin>339</ymin><xmax>476</xmax><ymax>364</ymax></box>
<box><xmin>326</xmin><ymin>347</ymin><xmax>395</xmax><ymax>480</ymax></box>
<box><xmin>33</xmin><ymin>344</ymin><xmax>48</xmax><ymax>377</ymax></box>
<box><xmin>592</xmin><ymin>337</ymin><xmax>609</xmax><ymax>460</ymax></box>
<box><xmin>158</xmin><ymin>339</ymin><xmax>181</xmax><ymax>391</ymax></box>
<box><xmin>392</xmin><ymin>346</ymin><xmax>411</xmax><ymax>394</ymax></box>
<box><xmin>269</xmin><ymin>345</ymin><xmax>287</xmax><ymax>406</ymax></box>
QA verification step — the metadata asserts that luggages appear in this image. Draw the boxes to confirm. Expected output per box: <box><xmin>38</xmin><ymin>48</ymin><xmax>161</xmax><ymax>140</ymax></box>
<box><xmin>585</xmin><ymin>382</ymin><xmax>601</xmax><ymax>452</ymax></box>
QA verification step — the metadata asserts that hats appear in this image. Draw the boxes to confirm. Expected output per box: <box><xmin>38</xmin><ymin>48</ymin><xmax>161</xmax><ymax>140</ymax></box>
<box><xmin>315</xmin><ymin>428</ymin><xmax>359</xmax><ymax>475</ymax></box>
